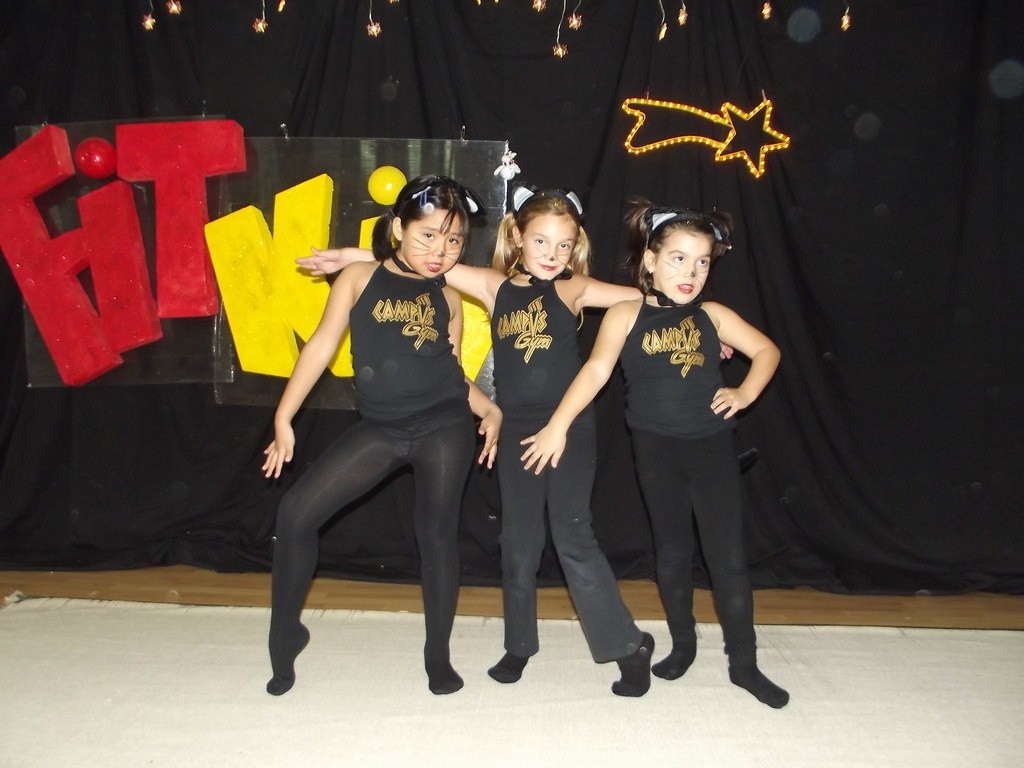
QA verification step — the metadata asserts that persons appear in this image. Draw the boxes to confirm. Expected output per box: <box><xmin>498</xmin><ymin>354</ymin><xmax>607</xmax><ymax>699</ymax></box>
<box><xmin>296</xmin><ymin>186</ymin><xmax>734</xmax><ymax>697</ymax></box>
<box><xmin>262</xmin><ymin>176</ymin><xmax>505</xmax><ymax>695</ymax></box>
<box><xmin>520</xmin><ymin>197</ymin><xmax>790</xmax><ymax>709</ymax></box>
